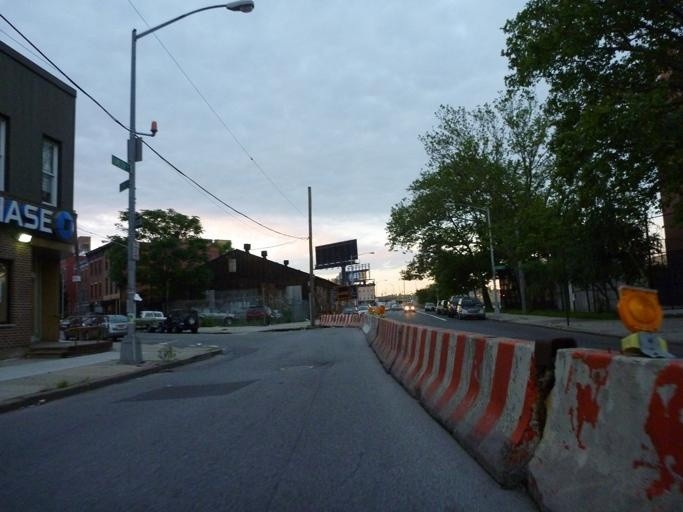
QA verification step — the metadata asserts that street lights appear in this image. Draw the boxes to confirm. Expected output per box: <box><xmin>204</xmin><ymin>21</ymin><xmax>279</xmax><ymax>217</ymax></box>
<box><xmin>446</xmin><ymin>201</ymin><xmax>501</xmax><ymax>319</ymax></box>
<box><xmin>122</xmin><ymin>1</ymin><xmax>255</xmax><ymax>364</ymax></box>
<box><xmin>351</xmin><ymin>251</ymin><xmax>375</xmax><ymax>307</ymax></box>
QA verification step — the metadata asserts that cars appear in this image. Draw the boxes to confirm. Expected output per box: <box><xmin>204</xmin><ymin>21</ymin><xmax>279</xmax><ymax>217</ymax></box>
<box><xmin>343</xmin><ymin>301</ymin><xmax>416</xmax><ymax>318</ymax></box>
<box><xmin>61</xmin><ymin>309</ymin><xmax>198</xmax><ymax>341</ymax></box>
<box><xmin>424</xmin><ymin>296</ymin><xmax>487</xmax><ymax>321</ymax></box>
<box><xmin>200</xmin><ymin>307</ymin><xmax>240</xmax><ymax>326</ymax></box>
<box><xmin>245</xmin><ymin>303</ymin><xmax>283</xmax><ymax>326</ymax></box>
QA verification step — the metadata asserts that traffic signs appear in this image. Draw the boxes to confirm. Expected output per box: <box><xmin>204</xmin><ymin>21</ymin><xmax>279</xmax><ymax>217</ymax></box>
<box><xmin>110</xmin><ymin>156</ymin><xmax>130</xmax><ymax>193</ymax></box>
